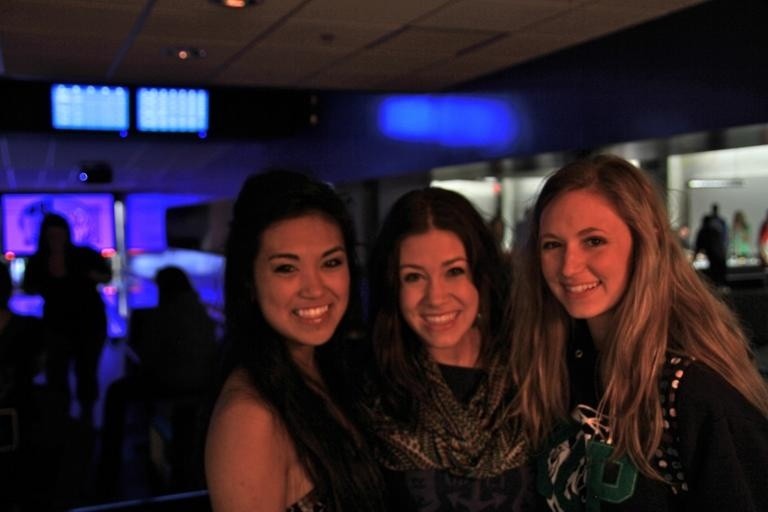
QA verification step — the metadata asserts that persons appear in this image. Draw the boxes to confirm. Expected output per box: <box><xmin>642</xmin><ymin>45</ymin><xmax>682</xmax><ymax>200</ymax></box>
<box><xmin>102</xmin><ymin>266</ymin><xmax>214</xmax><ymax>458</ymax></box>
<box><xmin>758</xmin><ymin>222</ymin><xmax>768</xmax><ymax>267</ymax></box>
<box><xmin>203</xmin><ymin>168</ymin><xmax>396</xmax><ymax>512</ymax></box>
<box><xmin>20</xmin><ymin>212</ymin><xmax>113</xmax><ymax>435</ymax></box>
<box><xmin>0</xmin><ymin>260</ymin><xmax>48</xmax><ymax>438</ymax></box>
<box><xmin>348</xmin><ymin>187</ymin><xmax>537</xmax><ymax>512</ymax></box>
<box><xmin>692</xmin><ymin>204</ymin><xmax>752</xmax><ymax>288</ymax></box>
<box><xmin>676</xmin><ymin>223</ymin><xmax>689</xmax><ymax>250</ymax></box>
<box><xmin>509</xmin><ymin>153</ymin><xmax>768</xmax><ymax>512</ymax></box>
